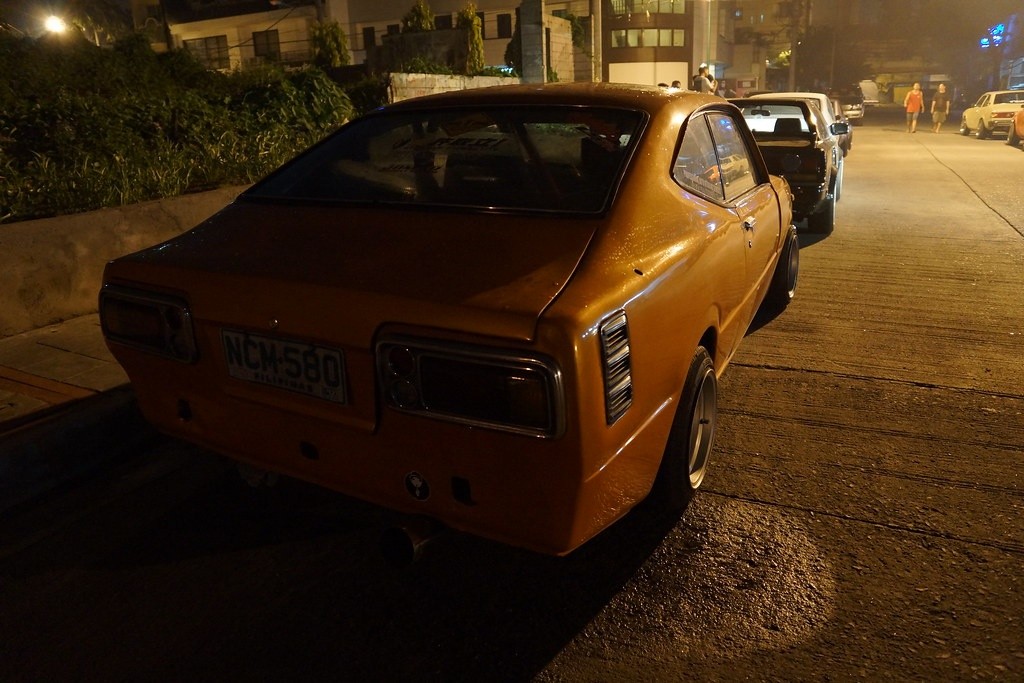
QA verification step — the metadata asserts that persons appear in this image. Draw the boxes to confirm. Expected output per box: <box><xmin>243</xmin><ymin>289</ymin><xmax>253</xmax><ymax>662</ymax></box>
<box><xmin>694</xmin><ymin>63</ymin><xmax>718</xmax><ymax>95</ymax></box>
<box><xmin>706</xmin><ymin>74</ymin><xmax>715</xmax><ymax>95</ymax></box>
<box><xmin>930</xmin><ymin>83</ymin><xmax>951</xmax><ymax>133</ymax></box>
<box><xmin>717</xmin><ymin>79</ymin><xmax>728</xmax><ymax>97</ymax></box>
<box><xmin>904</xmin><ymin>81</ymin><xmax>924</xmax><ymax>133</ymax></box>
<box><xmin>672</xmin><ymin>81</ymin><xmax>681</xmax><ymax>88</ymax></box>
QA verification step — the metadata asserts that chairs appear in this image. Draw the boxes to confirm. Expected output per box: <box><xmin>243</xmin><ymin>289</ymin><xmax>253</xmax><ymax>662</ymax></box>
<box><xmin>773</xmin><ymin>117</ymin><xmax>801</xmax><ymax>135</ymax></box>
<box><xmin>440</xmin><ymin>132</ymin><xmax>529</xmax><ymax>206</ymax></box>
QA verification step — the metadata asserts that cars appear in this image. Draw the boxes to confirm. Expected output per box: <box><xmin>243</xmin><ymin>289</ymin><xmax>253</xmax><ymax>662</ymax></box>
<box><xmin>841</xmin><ymin>94</ymin><xmax>867</xmax><ymax>126</ymax></box>
<box><xmin>1006</xmin><ymin>104</ymin><xmax>1024</xmax><ymax>148</ymax></box>
<box><xmin>96</xmin><ymin>81</ymin><xmax>806</xmax><ymax>566</ymax></box>
<box><xmin>959</xmin><ymin>87</ymin><xmax>1024</xmax><ymax>138</ymax></box>
<box><xmin>722</xmin><ymin>93</ymin><xmax>850</xmax><ymax>239</ymax></box>
